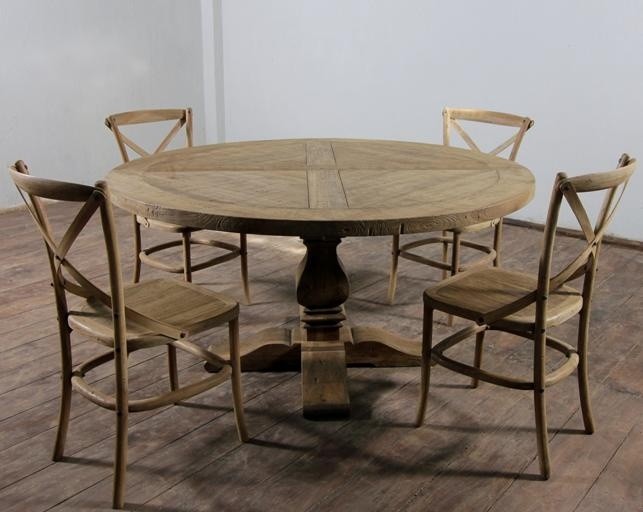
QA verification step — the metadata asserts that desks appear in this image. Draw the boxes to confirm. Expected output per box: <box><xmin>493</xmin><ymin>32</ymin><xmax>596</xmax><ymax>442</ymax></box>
<box><xmin>104</xmin><ymin>138</ymin><xmax>536</xmax><ymax>420</ymax></box>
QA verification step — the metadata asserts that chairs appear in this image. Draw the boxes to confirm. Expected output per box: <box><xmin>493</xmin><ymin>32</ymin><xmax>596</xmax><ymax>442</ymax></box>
<box><xmin>104</xmin><ymin>108</ymin><xmax>252</xmax><ymax>306</ymax></box>
<box><xmin>387</xmin><ymin>108</ymin><xmax>535</xmax><ymax>327</ymax></box>
<box><xmin>8</xmin><ymin>160</ymin><xmax>249</xmax><ymax>509</ymax></box>
<box><xmin>413</xmin><ymin>153</ymin><xmax>638</xmax><ymax>481</ymax></box>
